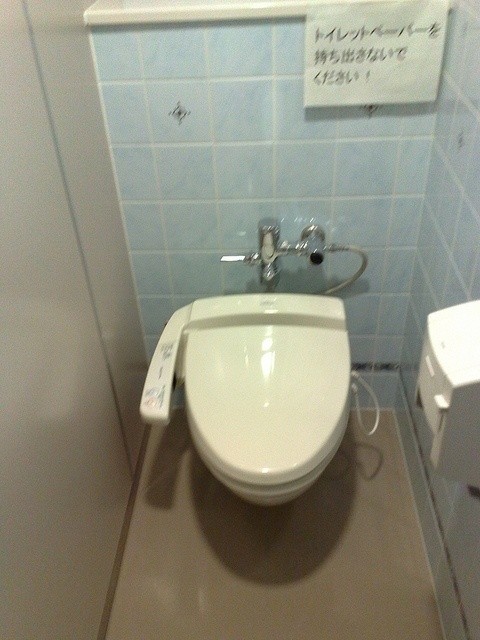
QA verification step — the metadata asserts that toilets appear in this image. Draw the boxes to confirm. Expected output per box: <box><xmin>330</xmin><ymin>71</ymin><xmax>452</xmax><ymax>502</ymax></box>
<box><xmin>185</xmin><ymin>293</ymin><xmax>352</xmax><ymax>507</ymax></box>
